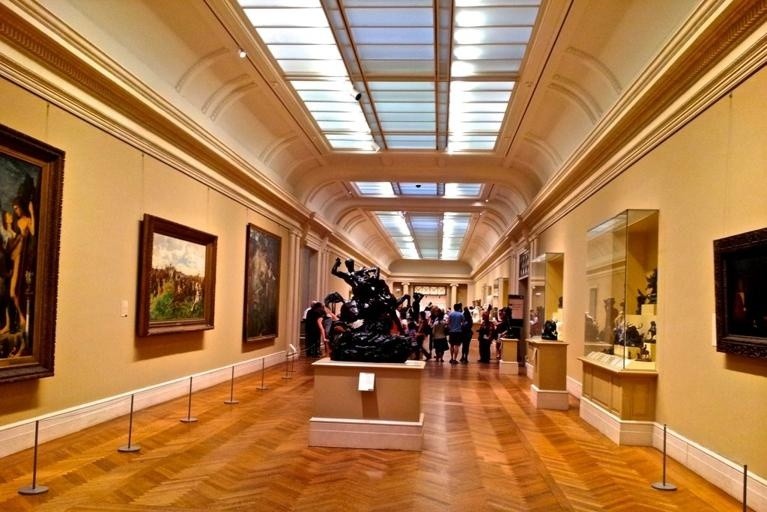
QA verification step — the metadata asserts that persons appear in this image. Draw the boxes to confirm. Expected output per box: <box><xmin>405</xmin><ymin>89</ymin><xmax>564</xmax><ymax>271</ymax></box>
<box><xmin>329</xmin><ymin>257</ymin><xmax>412</xmax><ymax>336</ymax></box>
<box><xmin>301</xmin><ymin>299</ymin><xmax>511</xmax><ymax>365</ymax></box>
<box><xmin>148</xmin><ymin>267</ymin><xmax>204</xmax><ymax>312</ymax></box>
<box><xmin>0</xmin><ymin>198</ymin><xmax>36</xmax><ymax>337</ymax></box>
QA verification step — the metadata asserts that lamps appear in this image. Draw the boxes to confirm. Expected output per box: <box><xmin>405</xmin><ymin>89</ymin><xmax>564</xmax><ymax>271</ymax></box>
<box><xmin>238</xmin><ymin>48</ymin><xmax>246</xmax><ymax>59</ymax></box>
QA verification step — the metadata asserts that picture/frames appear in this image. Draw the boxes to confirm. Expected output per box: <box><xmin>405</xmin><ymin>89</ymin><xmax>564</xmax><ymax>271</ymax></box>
<box><xmin>0</xmin><ymin>123</ymin><xmax>66</xmax><ymax>383</ymax></box>
<box><xmin>245</xmin><ymin>223</ymin><xmax>282</xmax><ymax>344</ymax></box>
<box><xmin>137</xmin><ymin>213</ymin><xmax>219</xmax><ymax>339</ymax></box>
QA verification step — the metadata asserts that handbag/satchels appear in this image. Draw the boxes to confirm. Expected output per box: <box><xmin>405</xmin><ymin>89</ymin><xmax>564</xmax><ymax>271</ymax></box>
<box><xmin>443</xmin><ymin>327</ymin><xmax>449</xmax><ymax>336</ymax></box>
<box><xmin>423</xmin><ymin>324</ymin><xmax>431</xmax><ymax>335</ymax></box>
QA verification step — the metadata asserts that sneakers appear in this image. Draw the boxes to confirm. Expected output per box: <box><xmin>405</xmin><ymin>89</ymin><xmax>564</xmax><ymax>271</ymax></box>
<box><xmin>460</xmin><ymin>359</ymin><xmax>468</xmax><ymax>363</ymax></box>
<box><xmin>434</xmin><ymin>356</ymin><xmax>444</xmax><ymax>362</ymax></box>
<box><xmin>449</xmin><ymin>360</ymin><xmax>458</xmax><ymax>364</ymax></box>
<box><xmin>424</xmin><ymin>356</ymin><xmax>431</xmax><ymax>361</ymax></box>
